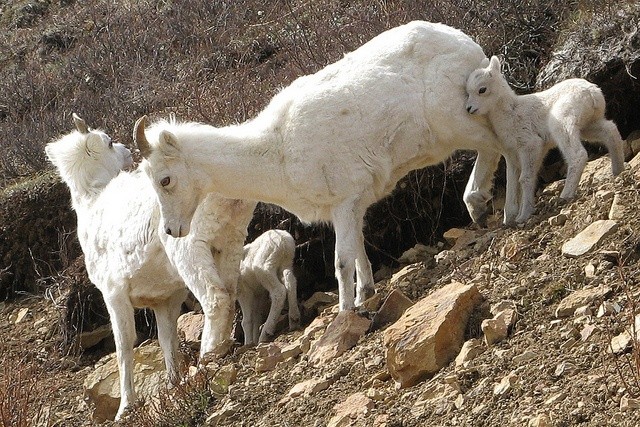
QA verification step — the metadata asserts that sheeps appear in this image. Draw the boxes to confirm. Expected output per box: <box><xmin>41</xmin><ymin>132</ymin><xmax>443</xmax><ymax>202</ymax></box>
<box><xmin>464</xmin><ymin>54</ymin><xmax>627</xmax><ymax>228</ymax></box>
<box><xmin>131</xmin><ymin>18</ymin><xmax>522</xmax><ymax>315</ymax></box>
<box><xmin>42</xmin><ymin>108</ymin><xmax>261</xmax><ymax>423</ymax></box>
<box><xmin>233</xmin><ymin>226</ymin><xmax>305</xmax><ymax>349</ymax></box>
